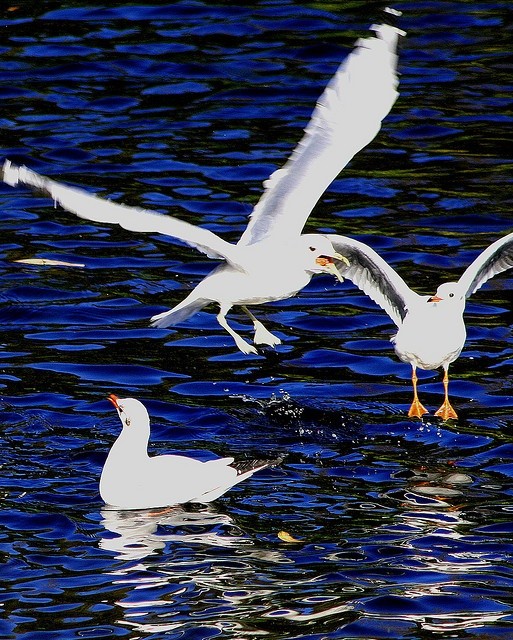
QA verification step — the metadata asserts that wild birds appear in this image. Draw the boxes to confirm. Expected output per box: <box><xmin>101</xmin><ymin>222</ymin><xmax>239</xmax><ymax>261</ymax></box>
<box><xmin>299</xmin><ymin>232</ymin><xmax>513</xmax><ymax>423</ymax></box>
<box><xmin>0</xmin><ymin>6</ymin><xmax>409</xmax><ymax>356</ymax></box>
<box><xmin>98</xmin><ymin>393</ymin><xmax>288</xmax><ymax>510</ymax></box>
